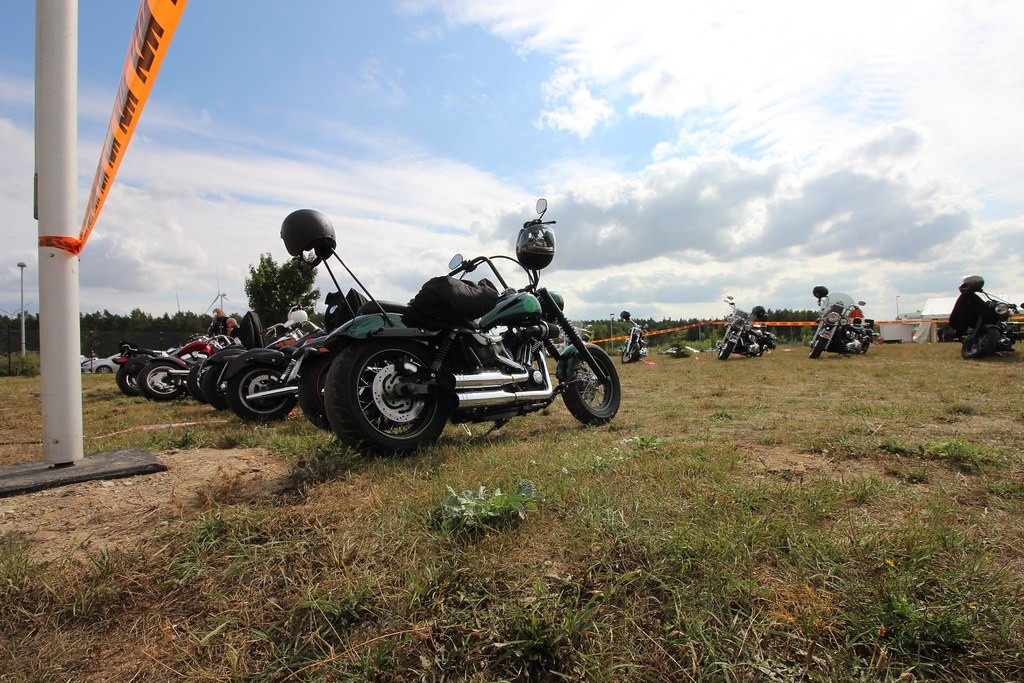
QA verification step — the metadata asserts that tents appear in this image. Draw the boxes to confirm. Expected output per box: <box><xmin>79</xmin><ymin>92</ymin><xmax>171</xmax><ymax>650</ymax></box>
<box><xmin>914</xmin><ymin>295</ymin><xmax>1024</xmax><ymax>343</ymax></box>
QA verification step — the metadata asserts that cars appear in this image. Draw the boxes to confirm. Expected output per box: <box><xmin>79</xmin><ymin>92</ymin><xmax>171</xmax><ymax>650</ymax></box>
<box><xmin>83</xmin><ymin>350</ymin><xmax>208</xmax><ymax>374</ymax></box>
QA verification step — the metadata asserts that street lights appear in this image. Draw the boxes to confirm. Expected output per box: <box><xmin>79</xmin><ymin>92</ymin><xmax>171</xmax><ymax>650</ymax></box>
<box><xmin>89</xmin><ymin>329</ymin><xmax>93</xmax><ymax>373</ymax></box>
<box><xmin>17</xmin><ymin>261</ymin><xmax>27</xmax><ymax>357</ymax></box>
<box><xmin>896</xmin><ymin>295</ymin><xmax>900</xmax><ymax>318</ymax></box>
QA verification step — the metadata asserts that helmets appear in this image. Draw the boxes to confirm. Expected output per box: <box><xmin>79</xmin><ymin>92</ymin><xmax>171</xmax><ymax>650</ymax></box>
<box><xmin>620</xmin><ymin>310</ymin><xmax>631</xmax><ymax>320</ymax></box>
<box><xmin>288</xmin><ymin>306</ymin><xmax>307</xmax><ymax>324</ymax></box>
<box><xmin>515</xmin><ymin>225</ymin><xmax>557</xmax><ymax>270</ymax></box>
<box><xmin>282</xmin><ymin>210</ymin><xmax>335</xmax><ymax>267</ymax></box>
<box><xmin>752</xmin><ymin>306</ymin><xmax>765</xmax><ymax>318</ymax></box>
<box><xmin>813</xmin><ymin>286</ymin><xmax>828</xmax><ymax>300</ymax></box>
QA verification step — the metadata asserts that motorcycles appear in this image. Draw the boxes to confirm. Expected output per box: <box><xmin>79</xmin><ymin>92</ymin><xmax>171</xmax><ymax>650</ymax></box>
<box><xmin>320</xmin><ymin>198</ymin><xmax>621</xmax><ymax>460</ymax></box>
<box><xmin>948</xmin><ymin>284</ymin><xmax>1018</xmax><ymax>362</ymax></box>
<box><xmin>808</xmin><ymin>292</ymin><xmax>880</xmax><ymax>358</ymax></box>
<box><xmin>112</xmin><ymin>289</ymin><xmax>417</xmax><ymax>434</ymax></box>
<box><xmin>620</xmin><ymin>318</ymin><xmax>651</xmax><ymax>364</ymax></box>
<box><xmin>716</xmin><ymin>295</ymin><xmax>778</xmax><ymax>361</ymax></box>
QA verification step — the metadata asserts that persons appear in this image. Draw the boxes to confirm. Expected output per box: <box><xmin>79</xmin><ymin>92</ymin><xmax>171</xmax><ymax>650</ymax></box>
<box><xmin>207</xmin><ymin>308</ymin><xmax>241</xmax><ymax>339</ymax></box>
